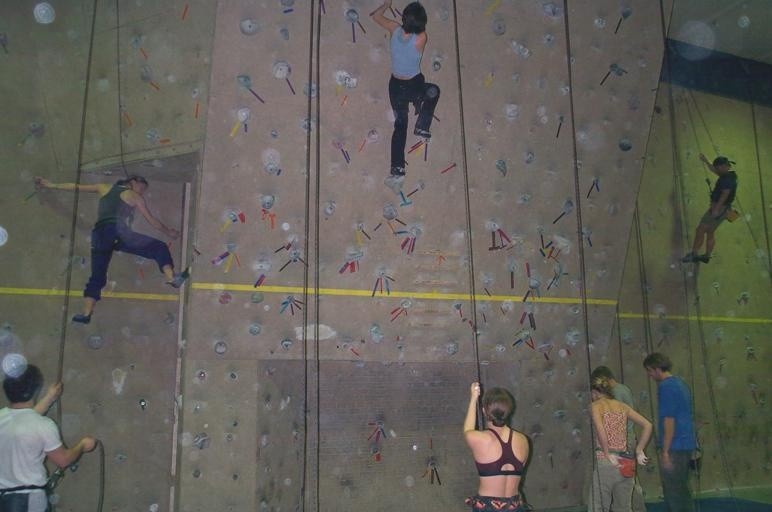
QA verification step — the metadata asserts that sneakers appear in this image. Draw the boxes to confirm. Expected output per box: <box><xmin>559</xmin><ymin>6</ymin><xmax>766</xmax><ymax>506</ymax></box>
<box><xmin>391</xmin><ymin>166</ymin><xmax>405</xmax><ymax>175</ymax></box>
<box><xmin>414</xmin><ymin>128</ymin><xmax>431</xmax><ymax>137</ymax></box>
<box><xmin>171</xmin><ymin>268</ymin><xmax>188</xmax><ymax>288</ymax></box>
<box><xmin>682</xmin><ymin>252</ymin><xmax>698</xmax><ymax>262</ymax></box>
<box><xmin>698</xmin><ymin>254</ymin><xmax>709</xmax><ymax>263</ymax></box>
<box><xmin>71</xmin><ymin>314</ymin><xmax>90</xmax><ymax>323</ymax></box>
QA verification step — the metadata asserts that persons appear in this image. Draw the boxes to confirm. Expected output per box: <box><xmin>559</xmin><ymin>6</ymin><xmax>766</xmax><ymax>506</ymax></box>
<box><xmin>585</xmin><ymin>375</ymin><xmax>653</xmax><ymax>511</ymax></box>
<box><xmin>371</xmin><ymin>1</ymin><xmax>440</xmax><ymax>177</ymax></box>
<box><xmin>679</xmin><ymin>153</ymin><xmax>738</xmax><ymax>264</ymax></box>
<box><xmin>33</xmin><ymin>172</ymin><xmax>194</xmax><ymax>325</ymax></box>
<box><xmin>33</xmin><ymin>380</ymin><xmax>64</xmax><ymax>415</ymax></box>
<box><xmin>643</xmin><ymin>352</ymin><xmax>699</xmax><ymax>512</ymax></box>
<box><xmin>591</xmin><ymin>366</ymin><xmax>646</xmax><ymax>512</ymax></box>
<box><xmin>462</xmin><ymin>380</ymin><xmax>536</xmax><ymax>511</ymax></box>
<box><xmin>2</xmin><ymin>360</ymin><xmax>99</xmax><ymax>511</ymax></box>
